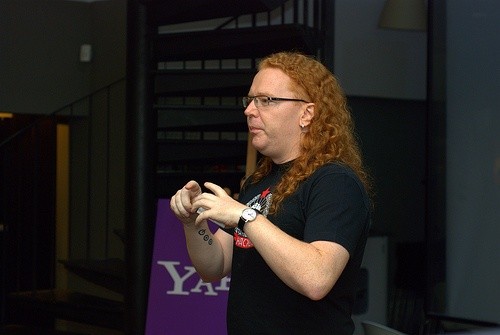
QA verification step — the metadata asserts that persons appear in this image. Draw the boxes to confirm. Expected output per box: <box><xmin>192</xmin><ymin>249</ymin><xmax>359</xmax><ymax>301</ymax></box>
<box><xmin>169</xmin><ymin>50</ymin><xmax>370</xmax><ymax>335</ymax></box>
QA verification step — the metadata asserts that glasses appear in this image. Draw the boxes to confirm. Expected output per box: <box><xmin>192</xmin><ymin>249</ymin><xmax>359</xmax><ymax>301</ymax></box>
<box><xmin>243</xmin><ymin>95</ymin><xmax>312</xmax><ymax>109</ymax></box>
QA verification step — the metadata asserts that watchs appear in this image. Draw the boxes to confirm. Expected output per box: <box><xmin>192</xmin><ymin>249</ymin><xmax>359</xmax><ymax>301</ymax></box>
<box><xmin>238</xmin><ymin>207</ymin><xmax>262</xmax><ymax>233</ymax></box>
